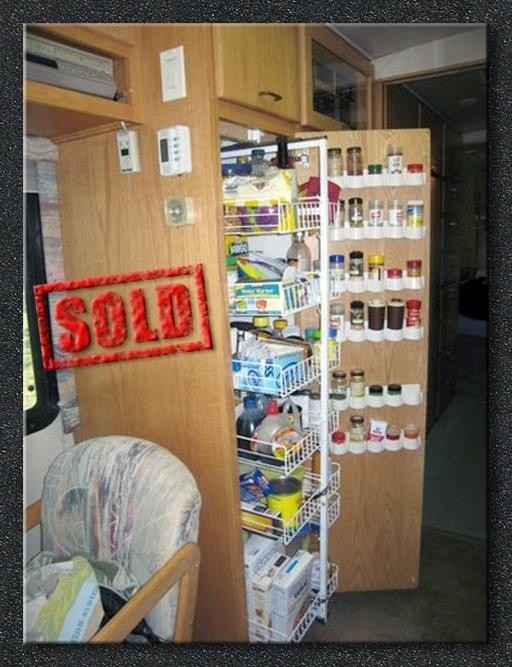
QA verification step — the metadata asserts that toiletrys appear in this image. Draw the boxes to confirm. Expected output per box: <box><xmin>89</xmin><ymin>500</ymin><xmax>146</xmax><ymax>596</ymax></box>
<box><xmin>287</xmin><ymin>233</ymin><xmax>313</xmax><ymax>273</ymax></box>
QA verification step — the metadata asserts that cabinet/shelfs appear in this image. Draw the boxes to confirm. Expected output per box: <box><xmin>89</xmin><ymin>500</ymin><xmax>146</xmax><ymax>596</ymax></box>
<box><xmin>22</xmin><ymin>24</ymin><xmax>462</xmax><ymax>642</ymax></box>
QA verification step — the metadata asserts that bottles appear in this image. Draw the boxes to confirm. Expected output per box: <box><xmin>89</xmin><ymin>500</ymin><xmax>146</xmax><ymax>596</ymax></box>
<box><xmin>235</xmin><ymin>136</ymin><xmax>424</xmax><ymax>468</ymax></box>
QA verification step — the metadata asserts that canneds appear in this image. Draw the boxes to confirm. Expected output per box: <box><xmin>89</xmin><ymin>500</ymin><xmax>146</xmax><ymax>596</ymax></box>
<box><xmin>267</xmin><ymin>476</ymin><xmax>304</xmax><ymax>534</ymax></box>
